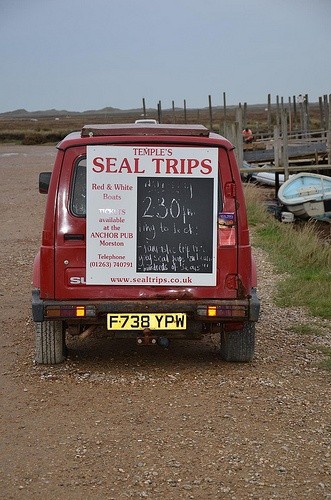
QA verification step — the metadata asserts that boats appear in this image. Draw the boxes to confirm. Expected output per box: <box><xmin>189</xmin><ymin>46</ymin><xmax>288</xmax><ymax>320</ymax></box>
<box><xmin>276</xmin><ymin>170</ymin><xmax>331</xmax><ymax>218</ymax></box>
<box><xmin>241</xmin><ymin>159</ymin><xmax>298</xmax><ymax>188</ymax></box>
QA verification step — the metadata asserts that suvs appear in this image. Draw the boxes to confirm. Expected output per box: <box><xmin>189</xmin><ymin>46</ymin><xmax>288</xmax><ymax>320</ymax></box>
<box><xmin>30</xmin><ymin>122</ymin><xmax>261</xmax><ymax>368</ymax></box>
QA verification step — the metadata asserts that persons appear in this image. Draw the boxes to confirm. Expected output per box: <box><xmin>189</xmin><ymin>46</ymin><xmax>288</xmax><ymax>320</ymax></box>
<box><xmin>242</xmin><ymin>127</ymin><xmax>254</xmax><ymax>143</ymax></box>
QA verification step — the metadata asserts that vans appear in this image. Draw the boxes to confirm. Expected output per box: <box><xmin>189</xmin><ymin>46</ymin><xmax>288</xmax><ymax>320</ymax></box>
<box><xmin>134</xmin><ymin>119</ymin><xmax>158</xmax><ymax>125</ymax></box>
